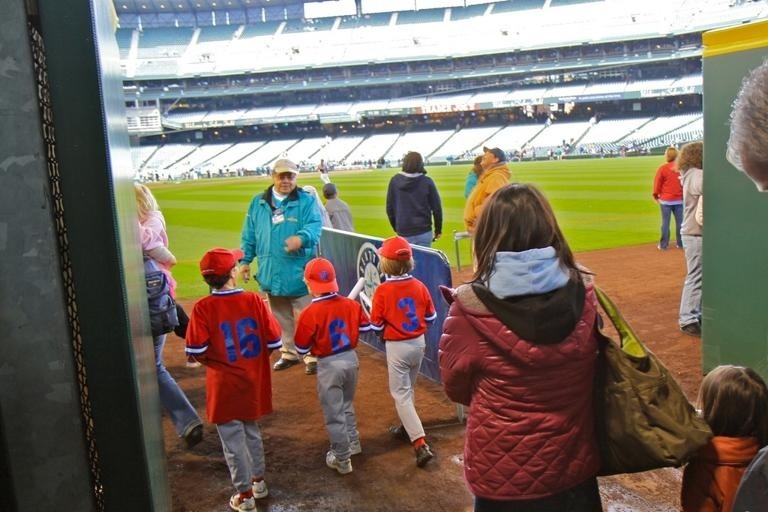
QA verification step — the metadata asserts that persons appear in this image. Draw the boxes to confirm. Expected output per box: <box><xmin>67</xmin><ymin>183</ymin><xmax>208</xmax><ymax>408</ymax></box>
<box><xmin>369</xmin><ymin>236</ymin><xmax>438</xmax><ymax>466</ymax></box>
<box><xmin>465</xmin><ymin>147</ymin><xmax>511</xmax><ymax>273</ymax></box>
<box><xmin>238</xmin><ymin>159</ymin><xmax>323</xmax><ymax>375</ymax></box>
<box><xmin>133</xmin><ymin>145</ymin><xmax>651</xmax><ymax>183</ymax></box>
<box><xmin>678</xmin><ymin>145</ymin><xmax>703</xmax><ymax>337</ymax></box>
<box><xmin>680</xmin><ymin>364</ymin><xmax>767</xmax><ymax>512</ymax></box>
<box><xmin>292</xmin><ymin>257</ymin><xmax>371</xmax><ymax>476</ymax></box>
<box><xmin>303</xmin><ymin>184</ymin><xmax>352</xmax><ymax>232</ymax></box>
<box><xmin>435</xmin><ymin>181</ymin><xmax>605</xmax><ymax>512</ymax></box>
<box><xmin>386</xmin><ymin>153</ymin><xmax>443</xmax><ymax>248</ymax></box>
<box><xmin>133</xmin><ymin>183</ymin><xmax>169</xmax><ymax>247</ymax></box>
<box><xmin>184</xmin><ymin>247</ymin><xmax>283</xmax><ymax>512</ymax></box>
<box><xmin>136</xmin><ymin>220</ymin><xmax>205</xmax><ymax>449</ymax></box>
<box><xmin>653</xmin><ymin>148</ymin><xmax>684</xmax><ymax>248</ymax></box>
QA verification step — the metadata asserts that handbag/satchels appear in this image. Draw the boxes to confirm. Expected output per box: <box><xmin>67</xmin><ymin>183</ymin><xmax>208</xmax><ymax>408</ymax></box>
<box><xmin>593</xmin><ymin>286</ymin><xmax>714</xmax><ymax>477</ymax></box>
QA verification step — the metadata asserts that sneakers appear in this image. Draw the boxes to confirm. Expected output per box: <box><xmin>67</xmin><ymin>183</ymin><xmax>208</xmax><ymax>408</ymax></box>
<box><xmin>680</xmin><ymin>322</ymin><xmax>701</xmax><ymax>336</ymax></box>
<box><xmin>186</xmin><ymin>428</ymin><xmax>204</xmax><ymax>450</ymax></box>
<box><xmin>229</xmin><ymin>490</ymin><xmax>257</xmax><ymax>511</ymax></box>
<box><xmin>304</xmin><ymin>362</ymin><xmax>315</xmax><ymax>375</ymax></box>
<box><xmin>349</xmin><ymin>441</ymin><xmax>363</xmax><ymax>455</ymax></box>
<box><xmin>251</xmin><ymin>479</ymin><xmax>268</xmax><ymax>499</ymax></box>
<box><xmin>416</xmin><ymin>444</ymin><xmax>432</xmax><ymax>467</ymax></box>
<box><xmin>325</xmin><ymin>451</ymin><xmax>353</xmax><ymax>474</ymax></box>
<box><xmin>388</xmin><ymin>422</ymin><xmax>406</xmax><ymax>440</ymax></box>
<box><xmin>272</xmin><ymin>359</ymin><xmax>299</xmax><ymax>371</ymax></box>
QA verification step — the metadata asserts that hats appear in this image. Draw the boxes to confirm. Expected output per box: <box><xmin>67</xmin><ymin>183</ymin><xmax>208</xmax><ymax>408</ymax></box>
<box><xmin>483</xmin><ymin>146</ymin><xmax>506</xmax><ymax>161</ymax></box>
<box><xmin>377</xmin><ymin>234</ymin><xmax>413</xmax><ymax>260</ymax></box>
<box><xmin>200</xmin><ymin>247</ymin><xmax>245</xmax><ymax>285</ymax></box>
<box><xmin>303</xmin><ymin>257</ymin><xmax>338</xmax><ymax>294</ymax></box>
<box><xmin>274</xmin><ymin>160</ymin><xmax>299</xmax><ymax>175</ymax></box>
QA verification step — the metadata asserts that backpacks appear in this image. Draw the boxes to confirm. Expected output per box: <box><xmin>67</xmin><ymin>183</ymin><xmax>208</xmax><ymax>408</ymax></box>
<box><xmin>136</xmin><ymin>253</ymin><xmax>180</xmax><ymax>336</ymax></box>
